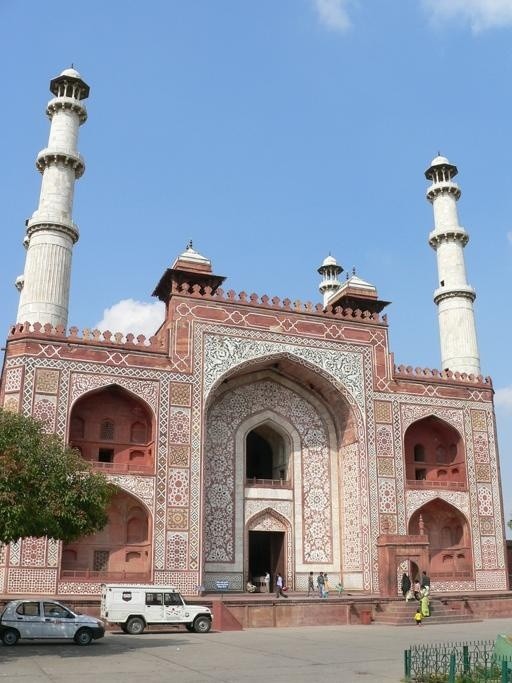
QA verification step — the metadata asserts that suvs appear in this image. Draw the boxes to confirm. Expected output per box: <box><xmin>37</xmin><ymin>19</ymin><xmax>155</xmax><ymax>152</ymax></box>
<box><xmin>100</xmin><ymin>583</ymin><xmax>214</xmax><ymax>634</ymax></box>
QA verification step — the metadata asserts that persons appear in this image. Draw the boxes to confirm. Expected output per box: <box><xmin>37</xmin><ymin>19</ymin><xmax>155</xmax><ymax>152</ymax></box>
<box><xmin>317</xmin><ymin>571</ymin><xmax>324</xmax><ymax>598</ymax></box>
<box><xmin>246</xmin><ymin>580</ymin><xmax>257</xmax><ymax>593</ymax></box>
<box><xmin>307</xmin><ymin>571</ymin><xmax>315</xmax><ymax>598</ymax></box>
<box><xmin>413</xmin><ymin>608</ymin><xmax>423</xmax><ymax>625</ymax></box>
<box><xmin>401</xmin><ymin>569</ymin><xmax>430</xmax><ymax>616</ymax></box>
<box><xmin>323</xmin><ymin>572</ymin><xmax>330</xmax><ymax>598</ymax></box>
<box><xmin>338</xmin><ymin>583</ymin><xmax>344</xmax><ymax>598</ymax></box>
<box><xmin>263</xmin><ymin>570</ymin><xmax>271</xmax><ymax>593</ymax></box>
<box><xmin>276</xmin><ymin>572</ymin><xmax>289</xmax><ymax>599</ymax></box>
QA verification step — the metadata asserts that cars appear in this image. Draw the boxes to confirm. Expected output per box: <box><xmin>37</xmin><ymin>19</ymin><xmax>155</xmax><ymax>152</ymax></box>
<box><xmin>0</xmin><ymin>599</ymin><xmax>105</xmax><ymax>646</ymax></box>
